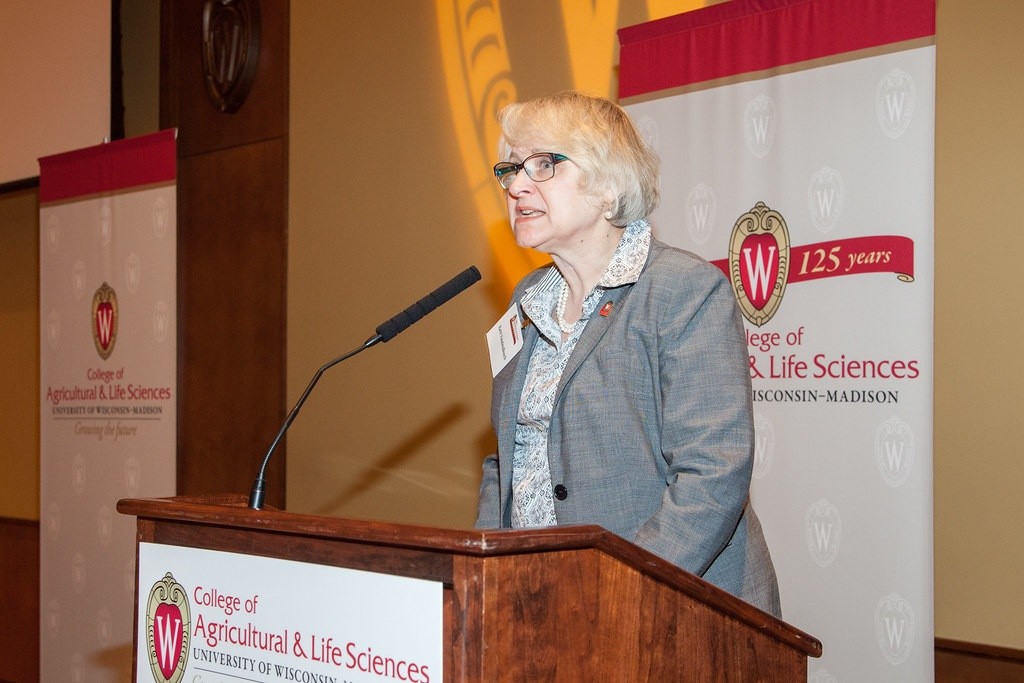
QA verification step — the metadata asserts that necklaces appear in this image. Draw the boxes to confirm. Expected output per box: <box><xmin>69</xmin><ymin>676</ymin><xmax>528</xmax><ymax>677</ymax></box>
<box><xmin>556</xmin><ymin>279</ymin><xmax>576</xmax><ymax>334</ymax></box>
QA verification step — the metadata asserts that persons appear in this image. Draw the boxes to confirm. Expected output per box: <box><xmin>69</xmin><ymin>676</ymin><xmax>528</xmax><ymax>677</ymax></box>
<box><xmin>474</xmin><ymin>94</ymin><xmax>782</xmax><ymax>622</ymax></box>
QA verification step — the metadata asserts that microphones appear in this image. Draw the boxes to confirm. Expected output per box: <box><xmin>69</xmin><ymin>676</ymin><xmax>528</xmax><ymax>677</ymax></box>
<box><xmin>248</xmin><ymin>266</ymin><xmax>483</xmax><ymax>507</ymax></box>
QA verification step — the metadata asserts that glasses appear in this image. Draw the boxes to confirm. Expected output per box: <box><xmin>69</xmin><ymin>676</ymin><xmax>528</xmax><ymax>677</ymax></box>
<box><xmin>493</xmin><ymin>152</ymin><xmax>570</xmax><ymax>190</ymax></box>
